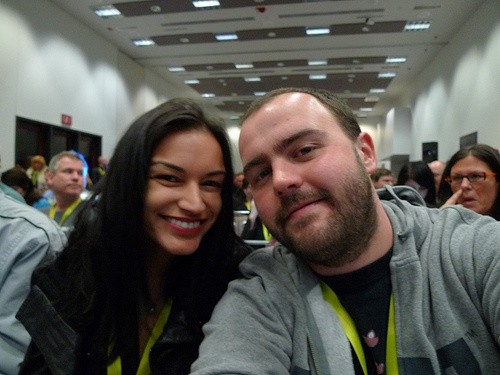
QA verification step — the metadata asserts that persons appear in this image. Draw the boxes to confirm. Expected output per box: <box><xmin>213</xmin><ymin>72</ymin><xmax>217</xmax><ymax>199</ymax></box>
<box><xmin>16</xmin><ymin>98</ymin><xmax>254</xmax><ymax>375</ymax></box>
<box><xmin>368</xmin><ymin>169</ymin><xmax>395</xmax><ymax>190</ymax></box>
<box><xmin>427</xmin><ymin>161</ymin><xmax>445</xmax><ymax>207</ymax></box>
<box><xmin>397</xmin><ymin>161</ymin><xmax>436</xmax><ymax>206</ymax></box>
<box><xmin>0</xmin><ymin>187</ymin><xmax>69</xmax><ymax>375</ymax></box>
<box><xmin>437</xmin><ymin>145</ymin><xmax>500</xmax><ymax>222</ymax></box>
<box><xmin>0</xmin><ymin>154</ymin><xmax>110</xmax><ymax>209</ymax></box>
<box><xmin>233</xmin><ymin>172</ymin><xmax>272</xmax><ymax>241</ymax></box>
<box><xmin>190</xmin><ymin>88</ymin><xmax>499</xmax><ymax>375</ymax></box>
<box><xmin>34</xmin><ymin>150</ymin><xmax>89</xmax><ymax>241</ymax></box>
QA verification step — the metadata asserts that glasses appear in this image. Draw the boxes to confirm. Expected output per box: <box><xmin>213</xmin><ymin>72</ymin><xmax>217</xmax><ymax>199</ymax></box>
<box><xmin>444</xmin><ymin>171</ymin><xmax>497</xmax><ymax>185</ymax></box>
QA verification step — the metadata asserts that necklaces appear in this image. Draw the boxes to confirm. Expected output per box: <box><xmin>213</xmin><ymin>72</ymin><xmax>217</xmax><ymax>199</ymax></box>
<box><xmin>49</xmin><ymin>196</ymin><xmax>81</xmax><ymax>225</ymax></box>
<box><xmin>318</xmin><ymin>280</ymin><xmax>397</xmax><ymax>374</ymax></box>
